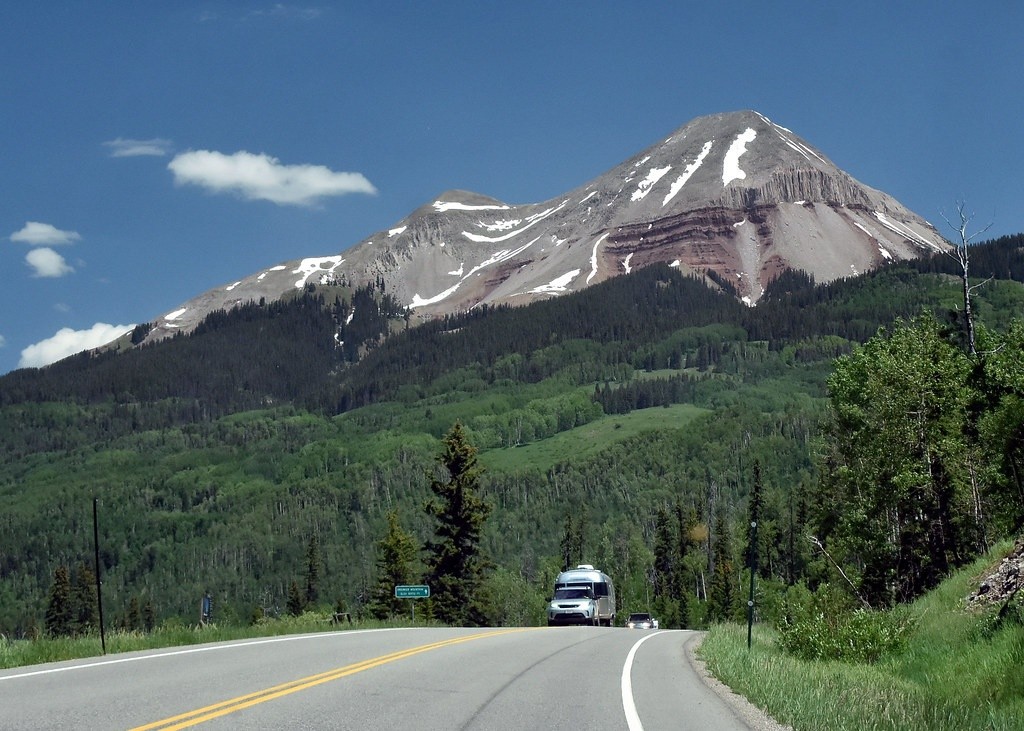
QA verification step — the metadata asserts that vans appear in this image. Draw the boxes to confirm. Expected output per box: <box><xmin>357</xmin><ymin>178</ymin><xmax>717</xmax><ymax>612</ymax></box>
<box><xmin>552</xmin><ymin>565</ymin><xmax>616</xmax><ymax>626</ymax></box>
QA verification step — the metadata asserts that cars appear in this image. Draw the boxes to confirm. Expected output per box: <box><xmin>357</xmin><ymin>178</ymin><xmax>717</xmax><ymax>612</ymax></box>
<box><xmin>624</xmin><ymin>612</ymin><xmax>654</xmax><ymax>629</ymax></box>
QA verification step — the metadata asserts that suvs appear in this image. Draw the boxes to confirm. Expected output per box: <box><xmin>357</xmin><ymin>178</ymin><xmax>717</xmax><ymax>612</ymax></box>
<box><xmin>545</xmin><ymin>585</ymin><xmax>601</xmax><ymax>626</ymax></box>
